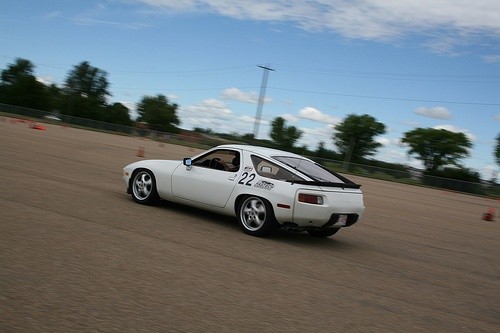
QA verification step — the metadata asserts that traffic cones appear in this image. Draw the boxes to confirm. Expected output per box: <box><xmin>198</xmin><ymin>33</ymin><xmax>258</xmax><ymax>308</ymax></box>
<box><xmin>481</xmin><ymin>204</ymin><xmax>493</xmax><ymax>220</ymax></box>
<box><xmin>137</xmin><ymin>140</ymin><xmax>144</xmax><ymax>158</ymax></box>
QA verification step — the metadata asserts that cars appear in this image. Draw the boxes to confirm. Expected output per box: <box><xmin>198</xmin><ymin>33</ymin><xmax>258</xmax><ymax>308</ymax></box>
<box><xmin>122</xmin><ymin>145</ymin><xmax>364</xmax><ymax>238</ymax></box>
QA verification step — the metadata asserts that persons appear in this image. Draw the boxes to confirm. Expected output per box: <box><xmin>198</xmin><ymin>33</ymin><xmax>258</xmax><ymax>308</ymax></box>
<box><xmin>216</xmin><ymin>153</ymin><xmax>240</xmax><ymax>172</ymax></box>
